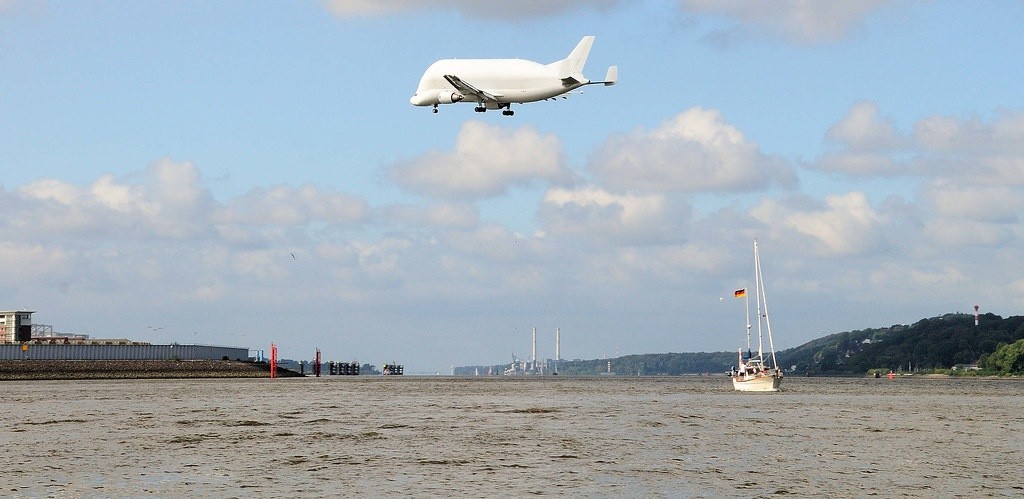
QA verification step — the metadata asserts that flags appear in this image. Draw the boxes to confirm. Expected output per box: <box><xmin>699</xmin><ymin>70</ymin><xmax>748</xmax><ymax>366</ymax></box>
<box><xmin>734</xmin><ymin>289</ymin><xmax>745</xmax><ymax>298</ymax></box>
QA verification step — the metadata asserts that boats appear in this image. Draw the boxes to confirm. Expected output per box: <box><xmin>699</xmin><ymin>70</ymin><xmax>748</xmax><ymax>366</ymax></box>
<box><xmin>503</xmin><ymin>360</ymin><xmax>548</xmax><ymax>377</ymax></box>
<box><xmin>724</xmin><ymin>365</ymin><xmax>739</xmax><ymax>376</ymax></box>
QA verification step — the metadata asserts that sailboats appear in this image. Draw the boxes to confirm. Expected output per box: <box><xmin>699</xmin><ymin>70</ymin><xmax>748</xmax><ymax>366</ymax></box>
<box><xmin>733</xmin><ymin>239</ymin><xmax>783</xmax><ymax>392</ymax></box>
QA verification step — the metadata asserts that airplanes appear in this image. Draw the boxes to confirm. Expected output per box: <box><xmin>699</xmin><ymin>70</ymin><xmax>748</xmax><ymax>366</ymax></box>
<box><xmin>408</xmin><ymin>36</ymin><xmax>618</xmax><ymax>118</ymax></box>
